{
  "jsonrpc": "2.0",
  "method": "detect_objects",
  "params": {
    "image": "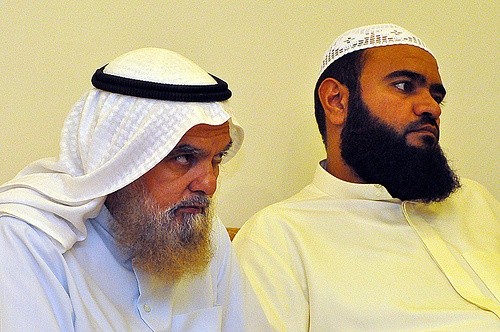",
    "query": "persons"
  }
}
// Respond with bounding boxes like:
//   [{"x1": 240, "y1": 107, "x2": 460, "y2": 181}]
[
  {"x1": 231, "y1": 23, "x2": 500, "y2": 332},
  {"x1": 0, "y1": 47, "x2": 277, "y2": 332}
]
